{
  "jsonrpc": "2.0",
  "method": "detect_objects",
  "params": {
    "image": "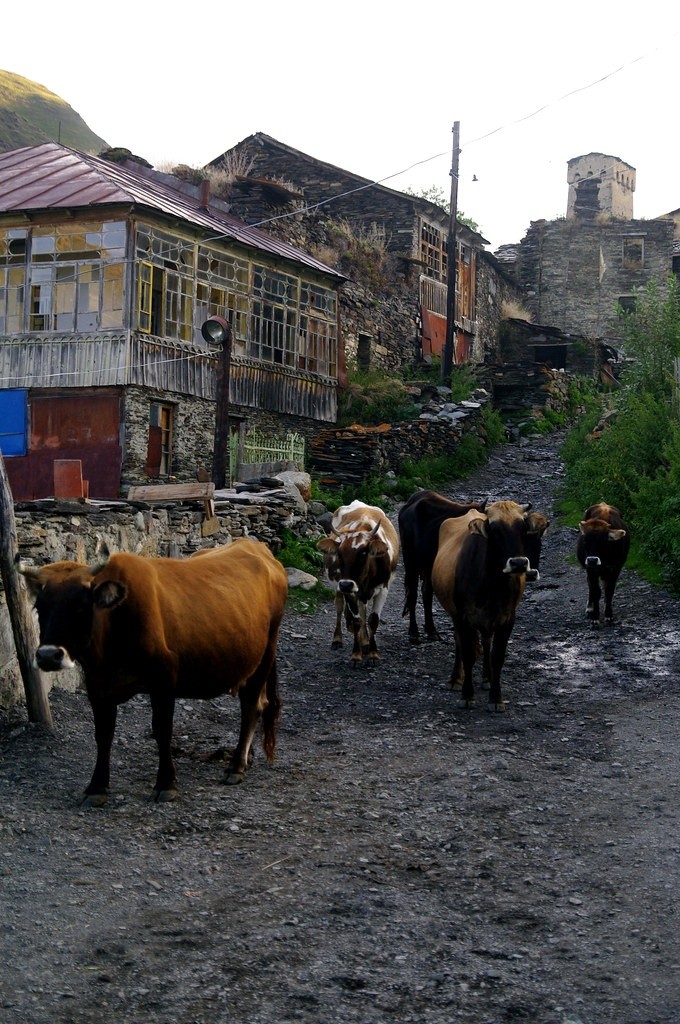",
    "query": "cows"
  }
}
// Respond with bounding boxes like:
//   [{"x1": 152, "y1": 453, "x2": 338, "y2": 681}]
[
  {"x1": 575, "y1": 501, "x2": 631, "y2": 626},
  {"x1": 397, "y1": 489, "x2": 551, "y2": 714},
  {"x1": 315, "y1": 498, "x2": 401, "y2": 669},
  {"x1": 13, "y1": 535, "x2": 290, "y2": 808}
]
